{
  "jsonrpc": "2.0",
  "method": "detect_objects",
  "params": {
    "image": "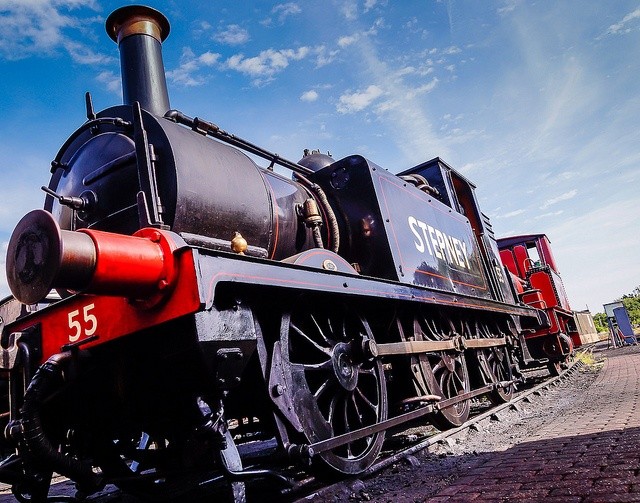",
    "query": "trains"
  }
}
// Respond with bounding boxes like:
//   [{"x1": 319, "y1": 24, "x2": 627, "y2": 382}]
[{"x1": 0, "y1": 3, "x2": 601, "y2": 501}]
